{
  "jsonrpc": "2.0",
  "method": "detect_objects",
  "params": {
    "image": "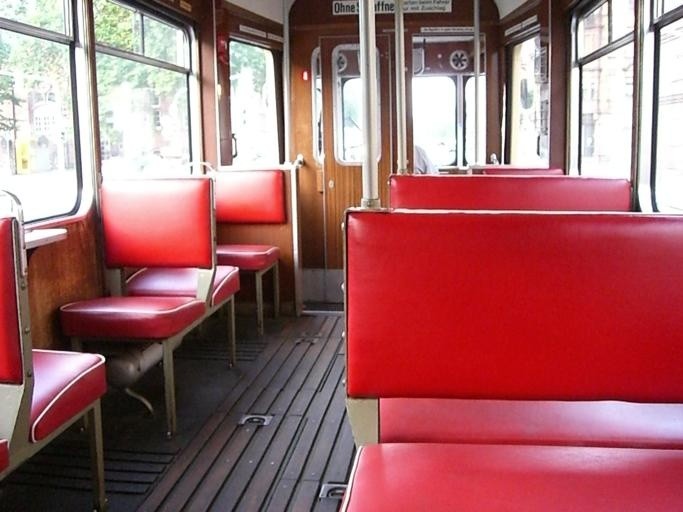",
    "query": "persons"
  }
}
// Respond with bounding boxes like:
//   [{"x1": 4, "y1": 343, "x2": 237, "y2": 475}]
[{"x1": 375, "y1": 126, "x2": 440, "y2": 177}]
